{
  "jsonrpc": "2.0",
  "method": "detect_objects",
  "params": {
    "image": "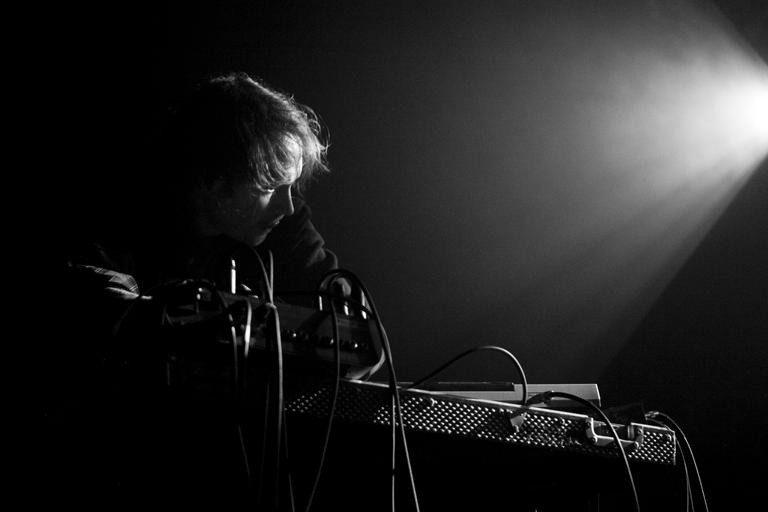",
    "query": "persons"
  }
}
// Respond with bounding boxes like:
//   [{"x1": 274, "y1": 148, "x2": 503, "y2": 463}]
[{"x1": 36, "y1": 72, "x2": 347, "y2": 315}]
